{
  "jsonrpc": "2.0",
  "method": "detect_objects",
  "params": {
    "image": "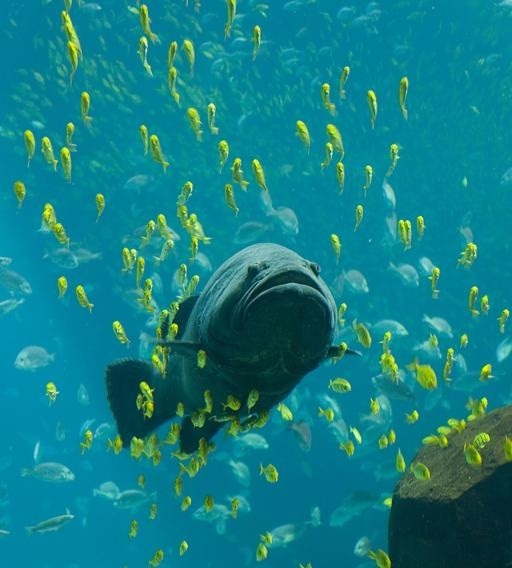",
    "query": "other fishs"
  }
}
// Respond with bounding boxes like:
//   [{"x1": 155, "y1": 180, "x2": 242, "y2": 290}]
[{"x1": 1, "y1": 0, "x2": 511, "y2": 568}]
